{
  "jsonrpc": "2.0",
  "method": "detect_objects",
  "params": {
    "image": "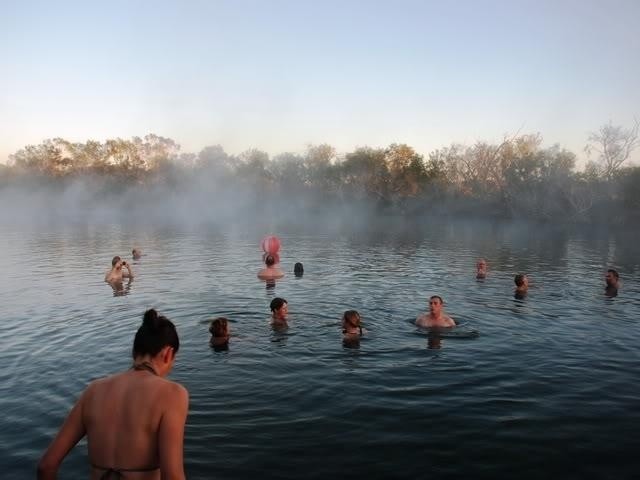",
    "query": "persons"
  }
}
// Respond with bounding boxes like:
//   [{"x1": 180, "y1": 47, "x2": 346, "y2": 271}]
[
  {"x1": 37, "y1": 309, "x2": 190, "y2": 479},
  {"x1": 209, "y1": 317, "x2": 230, "y2": 346},
  {"x1": 341, "y1": 310, "x2": 368, "y2": 335},
  {"x1": 257, "y1": 255, "x2": 284, "y2": 279},
  {"x1": 477, "y1": 259, "x2": 486, "y2": 277},
  {"x1": 133, "y1": 248, "x2": 140, "y2": 257},
  {"x1": 295, "y1": 263, "x2": 304, "y2": 277},
  {"x1": 514, "y1": 275, "x2": 529, "y2": 290},
  {"x1": 105, "y1": 256, "x2": 132, "y2": 283},
  {"x1": 606, "y1": 269, "x2": 622, "y2": 288},
  {"x1": 414, "y1": 295, "x2": 456, "y2": 327},
  {"x1": 270, "y1": 298, "x2": 289, "y2": 326}
]
